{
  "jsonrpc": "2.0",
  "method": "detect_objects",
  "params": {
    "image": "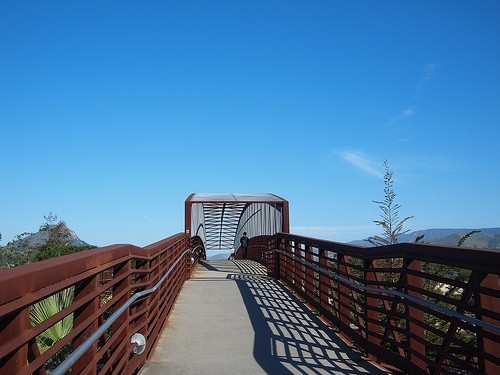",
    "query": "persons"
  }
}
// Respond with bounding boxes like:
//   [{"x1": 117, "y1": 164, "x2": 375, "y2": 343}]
[{"x1": 240, "y1": 232, "x2": 249, "y2": 260}]
